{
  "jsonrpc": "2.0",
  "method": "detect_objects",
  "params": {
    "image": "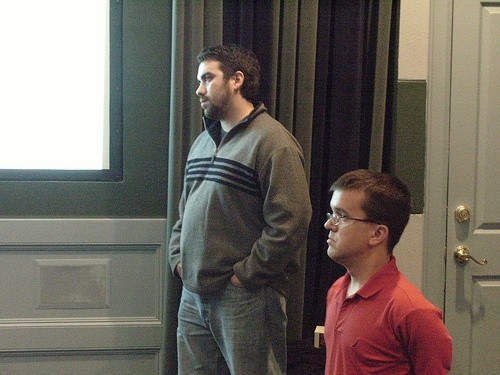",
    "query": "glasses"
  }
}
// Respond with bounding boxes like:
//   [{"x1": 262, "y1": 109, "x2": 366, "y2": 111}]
[{"x1": 327, "y1": 212, "x2": 382, "y2": 227}]
